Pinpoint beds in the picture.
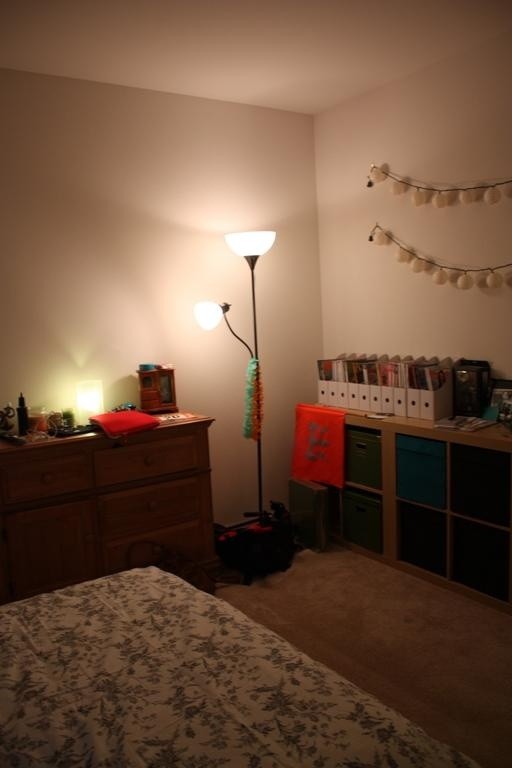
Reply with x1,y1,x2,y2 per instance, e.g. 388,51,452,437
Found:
1,564,480,767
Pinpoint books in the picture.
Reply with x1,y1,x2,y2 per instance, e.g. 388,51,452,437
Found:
317,358,512,432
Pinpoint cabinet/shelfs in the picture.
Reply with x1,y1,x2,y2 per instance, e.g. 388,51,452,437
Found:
312,403,512,615
0,410,216,601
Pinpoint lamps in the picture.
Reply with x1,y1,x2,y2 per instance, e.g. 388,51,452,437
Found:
195,228,276,518
